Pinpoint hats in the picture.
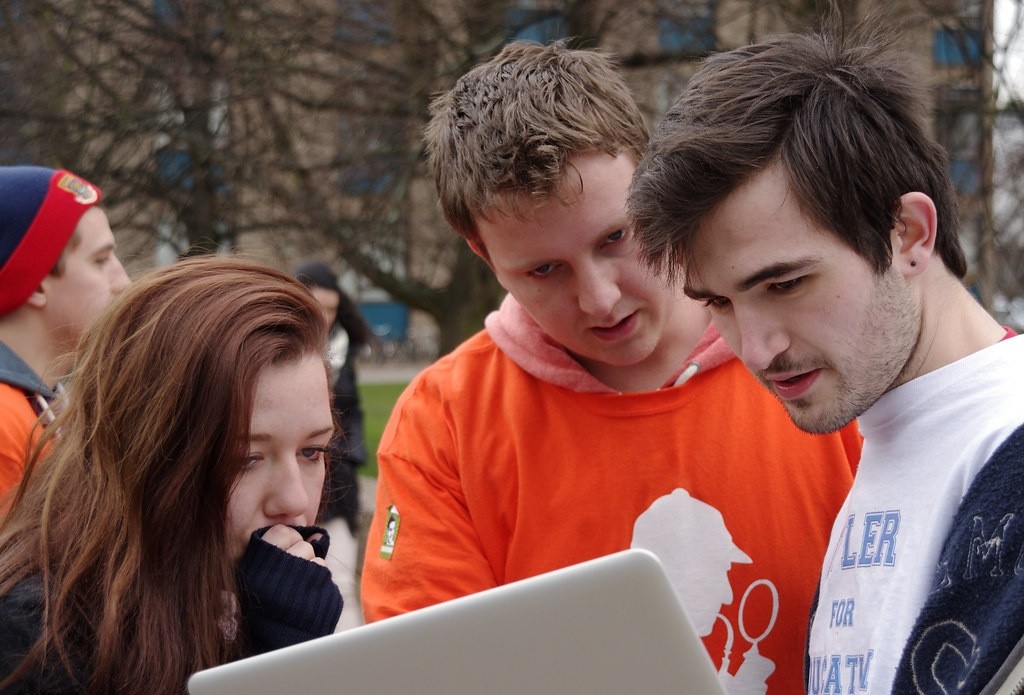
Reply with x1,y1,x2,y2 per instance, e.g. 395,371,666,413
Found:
0,164,104,317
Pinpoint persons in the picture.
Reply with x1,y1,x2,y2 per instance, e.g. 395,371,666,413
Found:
0,160,133,513
0,250,344,694
286,264,366,635
625,0,1024,695
355,43,862,695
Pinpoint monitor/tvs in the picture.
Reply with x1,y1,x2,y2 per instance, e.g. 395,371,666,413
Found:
186,546,728,695
